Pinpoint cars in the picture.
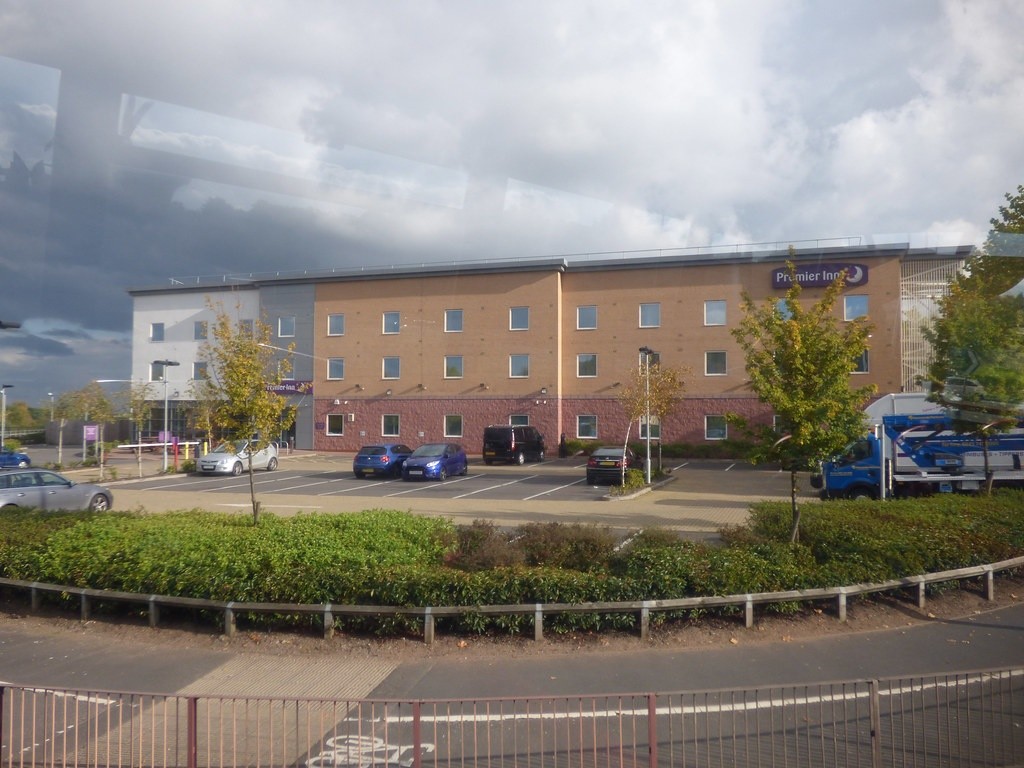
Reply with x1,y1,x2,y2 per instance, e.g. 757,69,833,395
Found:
402,441,468,482
0,468,114,516
0,446,31,470
195,439,279,478
353,442,414,480
587,444,643,486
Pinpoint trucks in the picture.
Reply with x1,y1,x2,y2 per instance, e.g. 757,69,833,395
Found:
854,392,954,427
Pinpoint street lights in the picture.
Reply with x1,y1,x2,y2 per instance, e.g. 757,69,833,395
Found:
1,384,15,446
47,392,54,422
639,346,653,484
153,359,181,472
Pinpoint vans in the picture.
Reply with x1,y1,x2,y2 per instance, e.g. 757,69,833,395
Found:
483,425,545,466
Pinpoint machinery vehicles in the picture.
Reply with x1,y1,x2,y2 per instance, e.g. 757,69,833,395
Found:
810,414,1024,503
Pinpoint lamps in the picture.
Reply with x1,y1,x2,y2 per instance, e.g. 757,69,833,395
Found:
417,383,423,388
355,384,362,388
541,388,547,394
386,389,392,395
334,399,340,405
480,382,486,388
